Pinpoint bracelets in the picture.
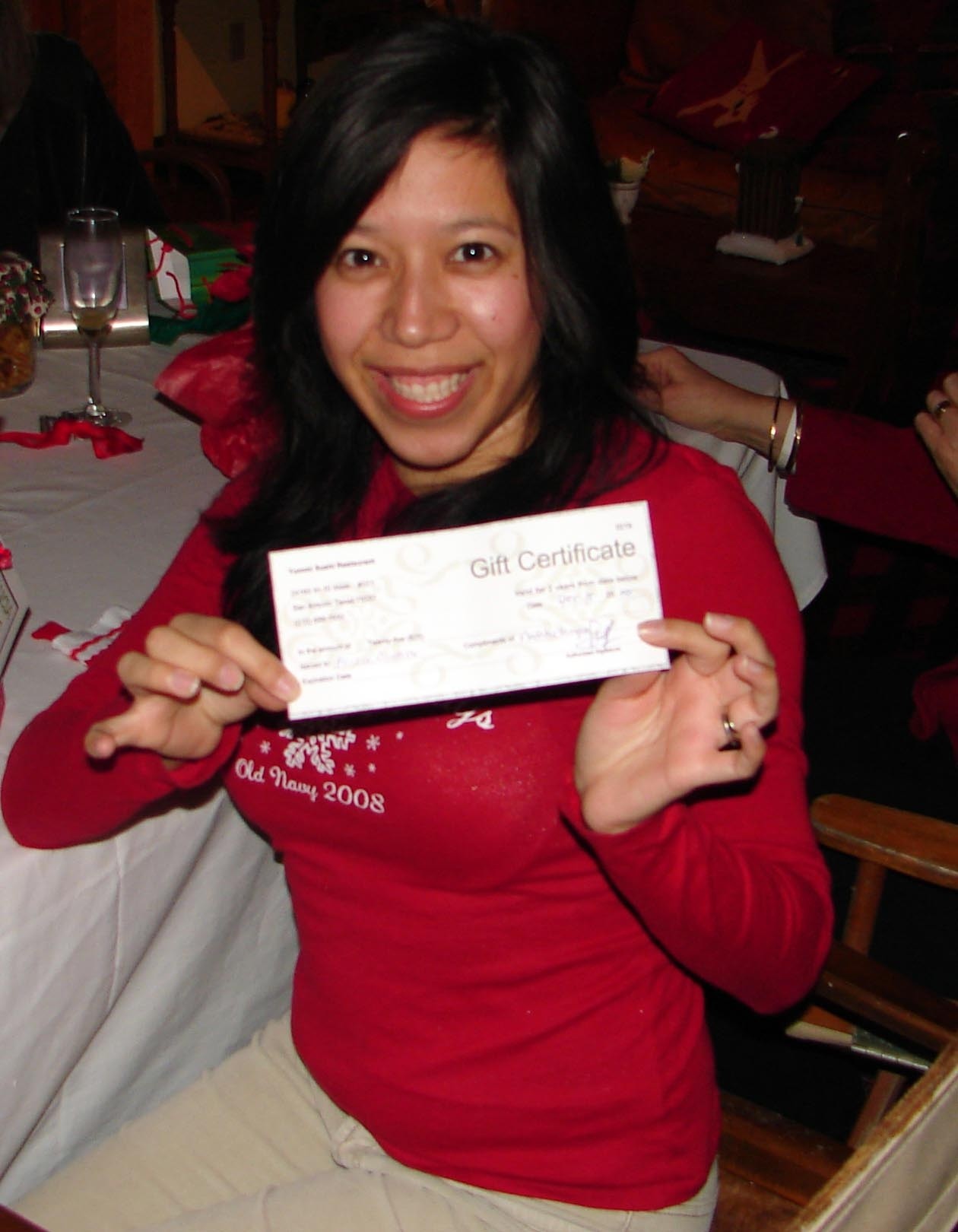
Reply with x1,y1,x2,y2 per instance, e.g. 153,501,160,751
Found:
768,397,781,472
778,402,803,479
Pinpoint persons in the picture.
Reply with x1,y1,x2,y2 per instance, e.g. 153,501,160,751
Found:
626,343,958,762
0,22,835,1232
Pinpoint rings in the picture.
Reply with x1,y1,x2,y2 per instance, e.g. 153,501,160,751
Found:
935,400,950,419
724,714,735,736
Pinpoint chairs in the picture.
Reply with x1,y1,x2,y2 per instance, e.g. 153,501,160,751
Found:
701,794,958,1232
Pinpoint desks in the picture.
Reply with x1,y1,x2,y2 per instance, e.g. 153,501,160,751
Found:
0,338,830,1204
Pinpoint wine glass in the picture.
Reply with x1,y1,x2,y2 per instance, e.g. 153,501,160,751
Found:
610,181,638,223
61,206,135,429
